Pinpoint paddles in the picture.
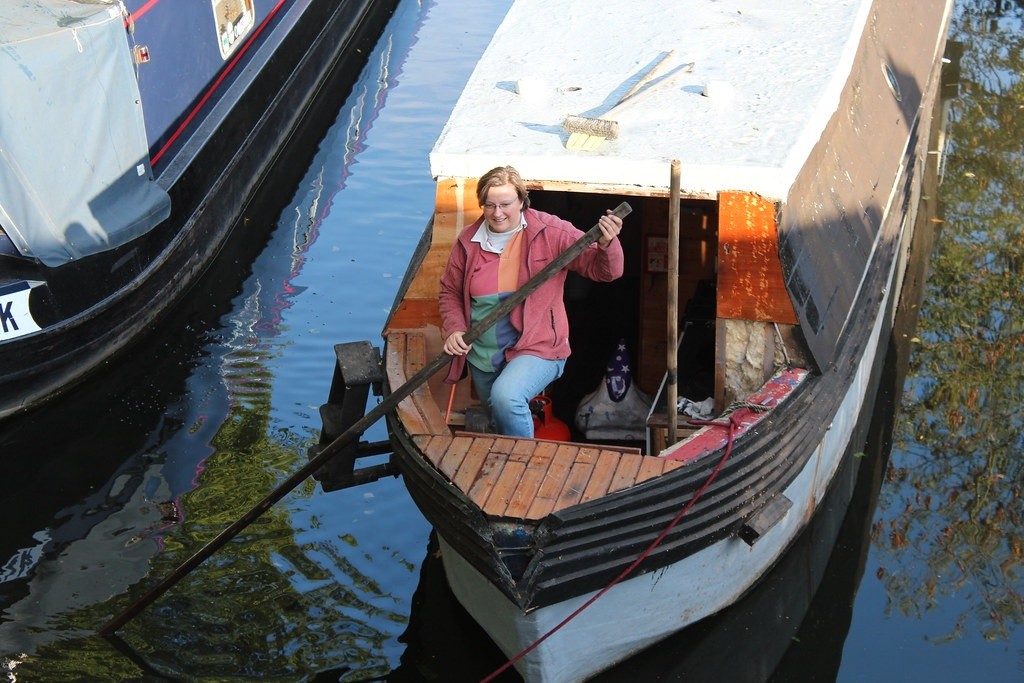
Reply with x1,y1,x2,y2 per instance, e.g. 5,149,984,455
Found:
101,200,633,632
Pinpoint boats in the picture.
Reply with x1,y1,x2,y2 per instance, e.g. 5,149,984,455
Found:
0,1,390,430
363,0,956,683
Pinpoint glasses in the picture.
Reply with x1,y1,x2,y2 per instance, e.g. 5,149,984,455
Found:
479,195,518,210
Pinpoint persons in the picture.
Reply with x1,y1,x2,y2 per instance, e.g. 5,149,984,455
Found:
438,167,624,438
